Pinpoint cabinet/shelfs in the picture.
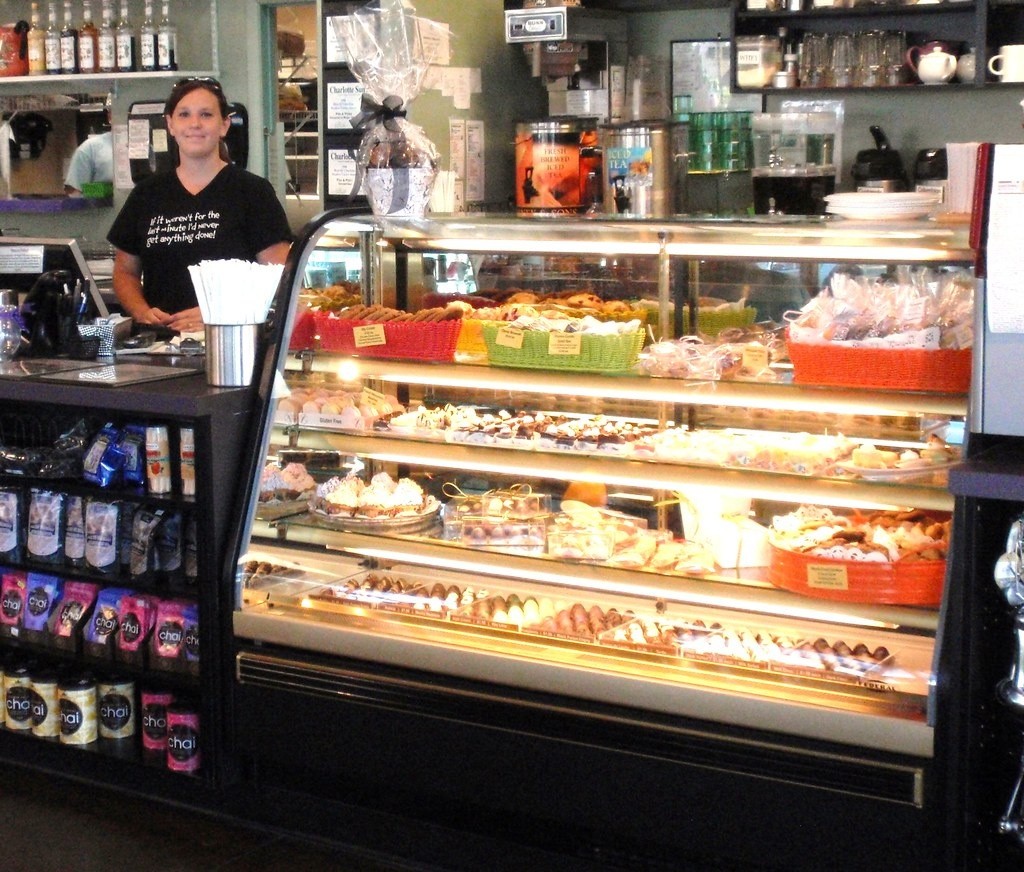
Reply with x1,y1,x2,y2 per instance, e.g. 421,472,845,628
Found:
728,0,1024,95
219,202,985,872
0,473,206,776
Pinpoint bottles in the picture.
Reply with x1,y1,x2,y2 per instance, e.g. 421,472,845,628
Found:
914,147,947,203
138,0,158,71
157,0,178,71
61,0,78,74
115,0,134,72
78,0,99,73
45,2,61,75
179,419,196,496
26,3,47,76
0,305,21,375
98,0,118,73
772,25,806,89
145,417,172,495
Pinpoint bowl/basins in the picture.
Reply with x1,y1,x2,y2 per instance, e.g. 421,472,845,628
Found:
81,240,119,261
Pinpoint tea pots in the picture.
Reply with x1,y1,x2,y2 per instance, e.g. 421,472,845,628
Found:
906,39,976,85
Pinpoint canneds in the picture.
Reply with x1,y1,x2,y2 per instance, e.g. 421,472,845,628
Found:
0,663,201,772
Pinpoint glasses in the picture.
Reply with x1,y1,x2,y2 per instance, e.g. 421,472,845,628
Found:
172,76,223,93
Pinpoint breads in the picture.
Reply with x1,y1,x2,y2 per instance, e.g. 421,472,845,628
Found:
442,300,569,321
503,293,631,313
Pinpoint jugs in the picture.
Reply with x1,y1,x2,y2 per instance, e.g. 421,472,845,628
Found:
0,20,30,77
850,125,909,193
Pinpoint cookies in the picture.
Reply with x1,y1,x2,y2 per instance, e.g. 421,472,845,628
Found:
340,304,465,325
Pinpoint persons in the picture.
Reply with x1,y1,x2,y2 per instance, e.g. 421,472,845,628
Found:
107,75,293,333
64,111,114,195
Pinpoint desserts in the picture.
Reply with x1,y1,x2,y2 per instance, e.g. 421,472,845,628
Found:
238,385,961,685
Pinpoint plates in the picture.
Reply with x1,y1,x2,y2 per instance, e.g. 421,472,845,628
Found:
824,192,938,222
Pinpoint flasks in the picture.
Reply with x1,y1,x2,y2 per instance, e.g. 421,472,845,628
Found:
0,288,19,304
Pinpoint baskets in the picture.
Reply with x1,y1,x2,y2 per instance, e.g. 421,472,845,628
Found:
313,308,462,362
482,319,646,373
650,306,758,342
456,315,513,355
767,536,946,606
783,326,971,393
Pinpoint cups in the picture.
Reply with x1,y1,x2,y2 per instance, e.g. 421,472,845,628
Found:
204,324,265,387
55,313,88,357
609,55,650,120
988,44,1024,82
802,31,908,86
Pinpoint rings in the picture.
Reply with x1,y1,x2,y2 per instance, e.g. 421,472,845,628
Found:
188,322,192,328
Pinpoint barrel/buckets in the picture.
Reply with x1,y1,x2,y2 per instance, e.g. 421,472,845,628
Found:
599,118,673,221
514,114,602,219
